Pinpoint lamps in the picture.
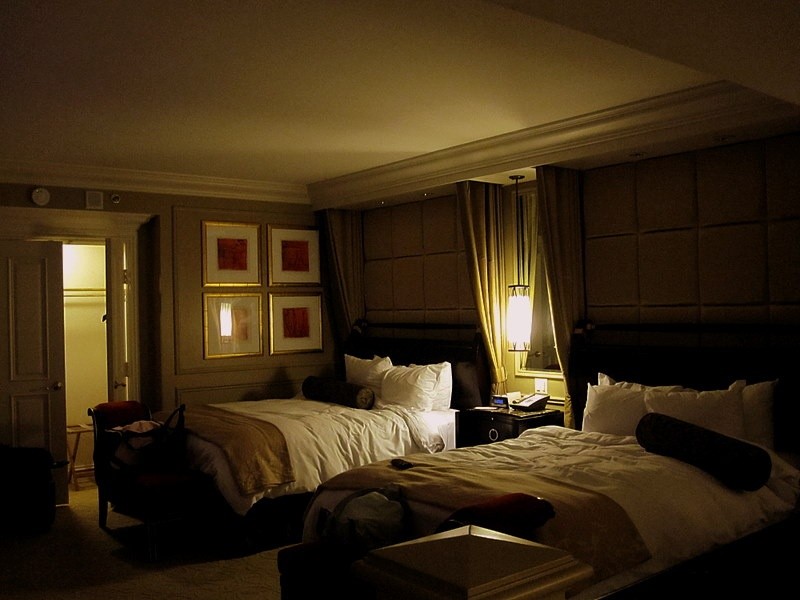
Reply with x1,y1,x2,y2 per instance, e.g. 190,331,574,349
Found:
508,175,533,352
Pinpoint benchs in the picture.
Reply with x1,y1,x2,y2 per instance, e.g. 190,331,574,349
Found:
279,494,556,600
88,400,194,536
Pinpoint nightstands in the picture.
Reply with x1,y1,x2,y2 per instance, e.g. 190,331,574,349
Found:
455,406,564,448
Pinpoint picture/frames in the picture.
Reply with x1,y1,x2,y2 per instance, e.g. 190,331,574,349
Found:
203,292,263,360
266,223,323,287
202,220,263,287
268,292,325,356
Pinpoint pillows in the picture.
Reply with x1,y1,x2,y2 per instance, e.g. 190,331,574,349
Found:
301,354,453,409
580,372,780,492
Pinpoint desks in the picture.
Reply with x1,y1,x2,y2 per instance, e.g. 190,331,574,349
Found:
67,423,94,491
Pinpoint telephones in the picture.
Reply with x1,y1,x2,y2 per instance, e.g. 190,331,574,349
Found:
510,393,550,412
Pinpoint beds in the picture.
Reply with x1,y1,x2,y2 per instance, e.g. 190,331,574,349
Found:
151,323,491,564
301,319,800,600
